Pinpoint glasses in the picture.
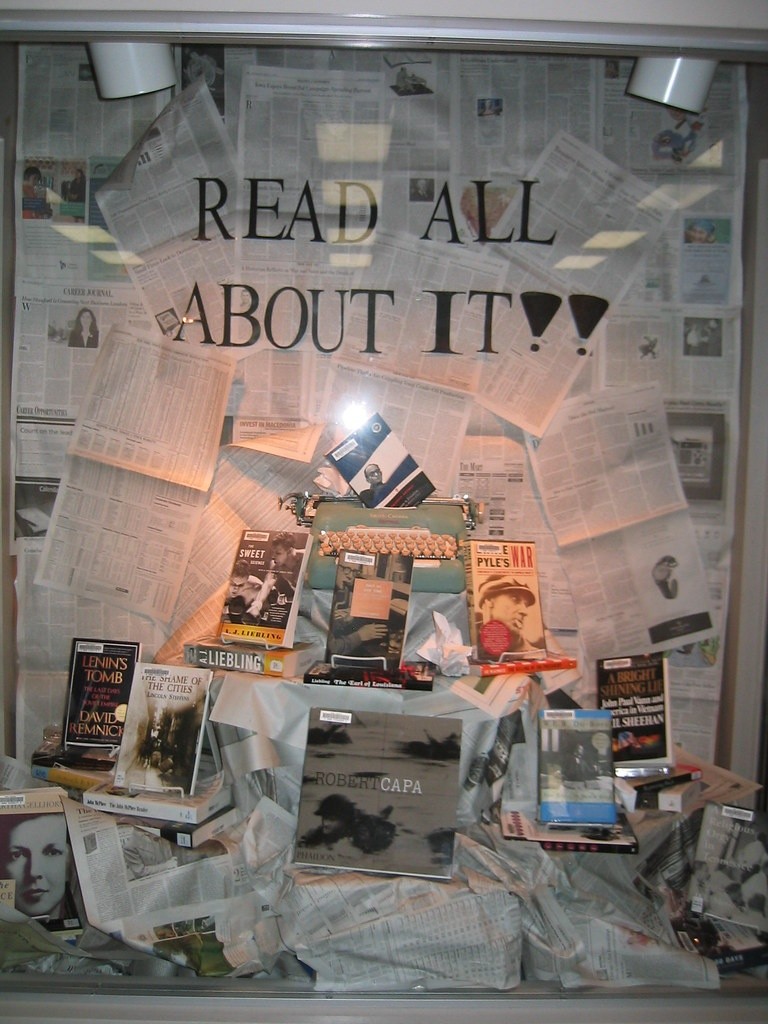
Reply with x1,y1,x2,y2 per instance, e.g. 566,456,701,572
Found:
339,563,361,578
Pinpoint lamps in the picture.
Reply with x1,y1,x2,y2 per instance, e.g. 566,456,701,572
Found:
626,58,722,115
90,42,179,102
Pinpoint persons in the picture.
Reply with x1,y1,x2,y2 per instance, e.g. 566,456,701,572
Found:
0,812,84,933
326,550,389,666
475,572,541,662
222,561,270,629
564,743,597,782
359,463,393,508
240,531,306,626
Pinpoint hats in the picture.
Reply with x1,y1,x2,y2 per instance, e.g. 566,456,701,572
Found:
478,572,535,608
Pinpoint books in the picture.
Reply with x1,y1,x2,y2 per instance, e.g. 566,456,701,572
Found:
302,547,440,693
180,528,328,681
499,708,641,857
594,649,705,816
667,799,768,976
290,705,464,887
0,786,84,946
30,636,239,852
460,538,580,678
324,410,437,511
